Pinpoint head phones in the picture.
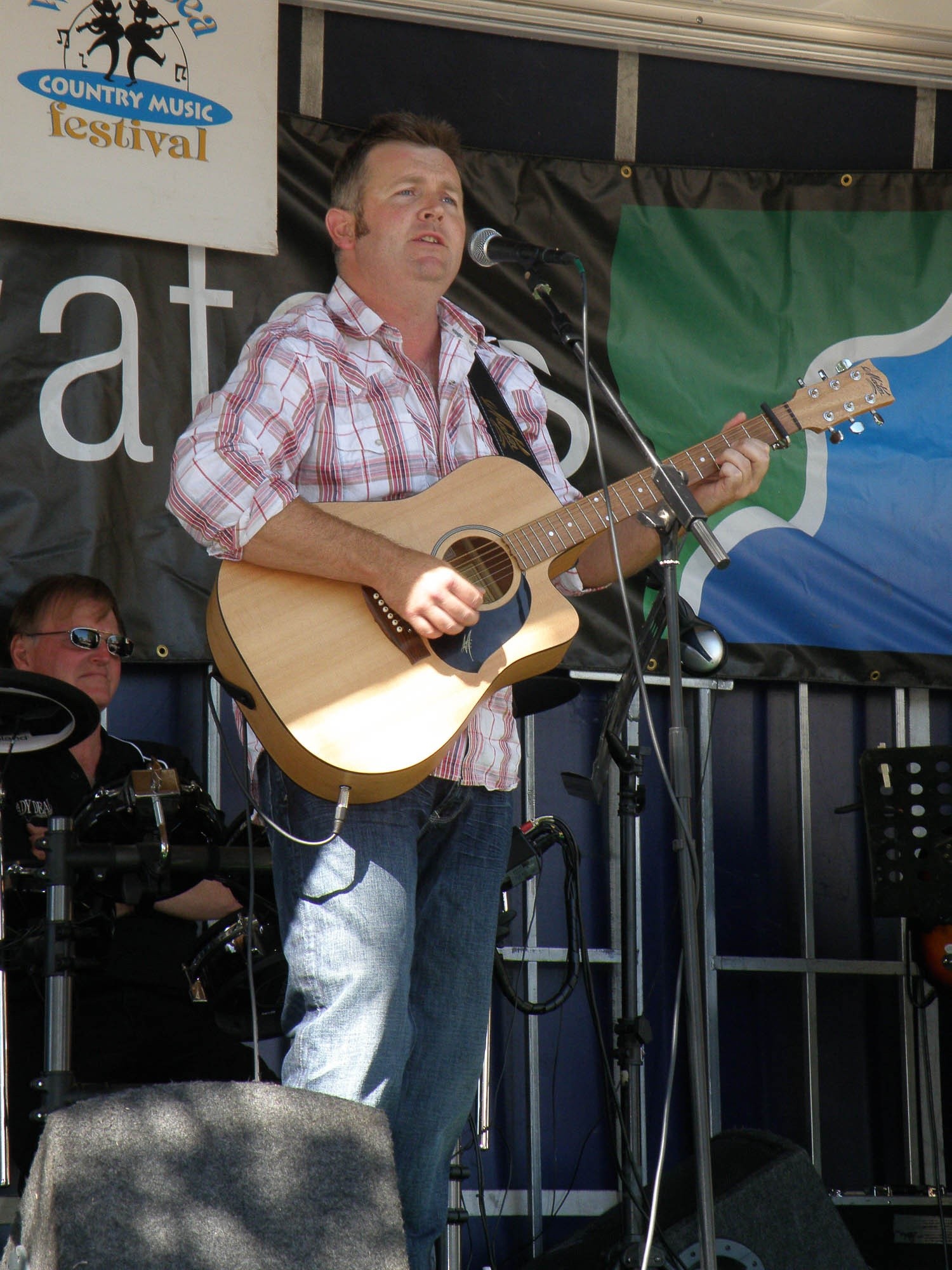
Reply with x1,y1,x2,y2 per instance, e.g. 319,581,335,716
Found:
645,559,730,675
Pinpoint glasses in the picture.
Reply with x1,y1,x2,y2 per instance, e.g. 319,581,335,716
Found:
23,627,135,658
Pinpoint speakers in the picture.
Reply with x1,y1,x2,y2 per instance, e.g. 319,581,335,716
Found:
578,1129,873,1270
2,1082,412,1270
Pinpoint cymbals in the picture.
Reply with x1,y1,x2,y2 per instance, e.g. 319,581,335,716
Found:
0,667,101,760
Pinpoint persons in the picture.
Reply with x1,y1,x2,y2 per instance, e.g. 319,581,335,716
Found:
166,110,773,1270
1,572,256,1090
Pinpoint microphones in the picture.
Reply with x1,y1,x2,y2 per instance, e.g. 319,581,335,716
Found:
466,227,580,267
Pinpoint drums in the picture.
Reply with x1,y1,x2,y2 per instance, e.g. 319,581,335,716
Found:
68,778,221,904
183,903,287,1041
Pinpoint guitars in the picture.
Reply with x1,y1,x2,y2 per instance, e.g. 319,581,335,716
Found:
205,358,896,804
